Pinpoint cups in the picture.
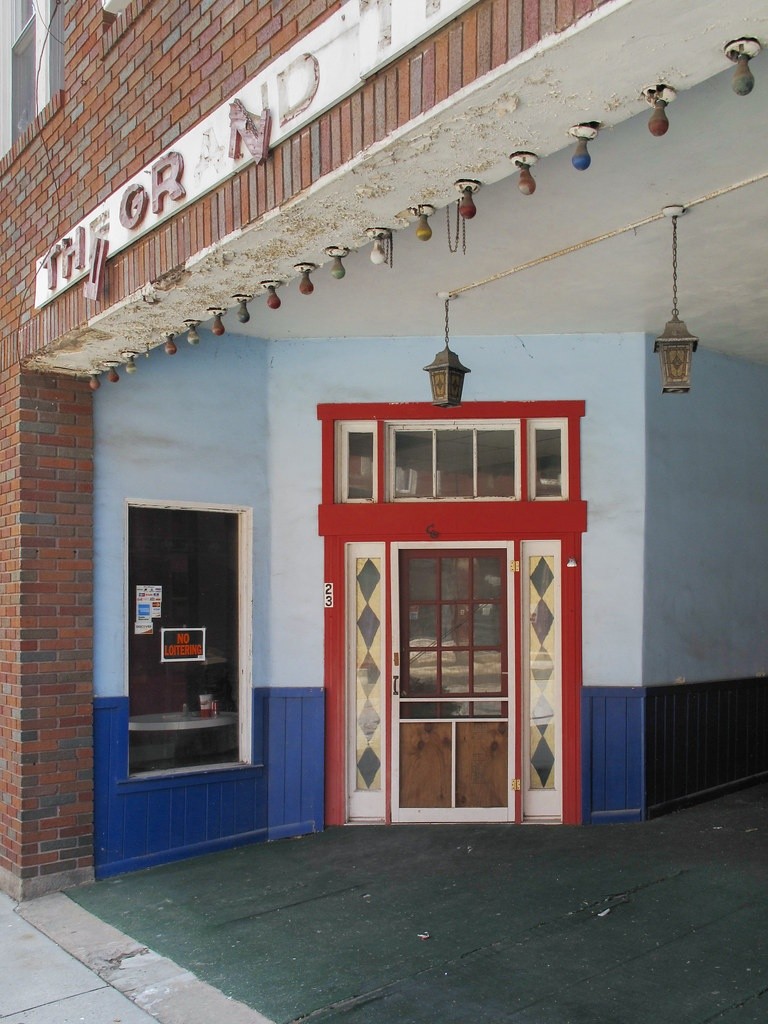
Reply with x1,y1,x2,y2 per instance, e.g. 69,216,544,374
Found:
199,694,213,718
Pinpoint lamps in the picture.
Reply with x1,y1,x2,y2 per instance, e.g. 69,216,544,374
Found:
422,290,472,408
653,205,699,394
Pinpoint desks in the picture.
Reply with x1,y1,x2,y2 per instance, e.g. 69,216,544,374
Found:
129,711,238,770
